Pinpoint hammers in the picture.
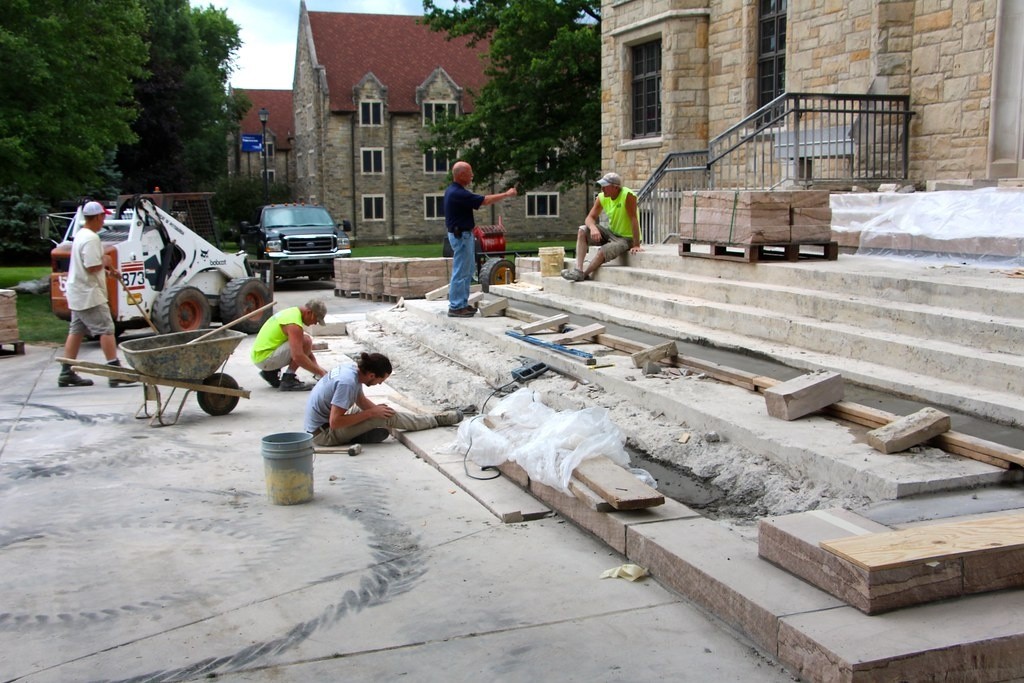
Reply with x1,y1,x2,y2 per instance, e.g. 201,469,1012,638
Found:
313,444,362,456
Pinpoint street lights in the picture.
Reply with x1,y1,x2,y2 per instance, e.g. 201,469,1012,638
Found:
257,107,270,200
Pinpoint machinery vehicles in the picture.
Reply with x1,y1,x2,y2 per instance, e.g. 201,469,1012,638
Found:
39,186,275,342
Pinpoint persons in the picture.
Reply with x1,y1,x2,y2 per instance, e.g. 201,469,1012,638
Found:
444,161,518,318
57,202,136,387
562,172,643,282
306,352,463,447
251,297,327,391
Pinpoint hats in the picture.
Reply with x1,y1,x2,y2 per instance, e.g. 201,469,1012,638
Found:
305,299,327,326
83,201,113,215
597,173,621,187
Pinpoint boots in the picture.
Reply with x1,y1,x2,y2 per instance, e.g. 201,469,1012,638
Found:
58,363,94,387
107,360,137,387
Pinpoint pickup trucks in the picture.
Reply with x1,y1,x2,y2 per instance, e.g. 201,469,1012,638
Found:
240,203,353,285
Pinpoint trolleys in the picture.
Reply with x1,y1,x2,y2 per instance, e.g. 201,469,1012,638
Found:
54,326,253,427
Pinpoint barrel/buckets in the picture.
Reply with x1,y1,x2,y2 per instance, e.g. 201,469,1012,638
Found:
260,432,317,504
538,246,565,277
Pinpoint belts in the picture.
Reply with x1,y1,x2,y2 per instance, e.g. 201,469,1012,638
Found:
311,422,330,439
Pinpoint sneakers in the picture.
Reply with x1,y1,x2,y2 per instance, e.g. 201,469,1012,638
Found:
560,267,584,281
447,305,478,317
352,428,389,443
433,410,464,426
259,369,281,388
279,373,312,391
